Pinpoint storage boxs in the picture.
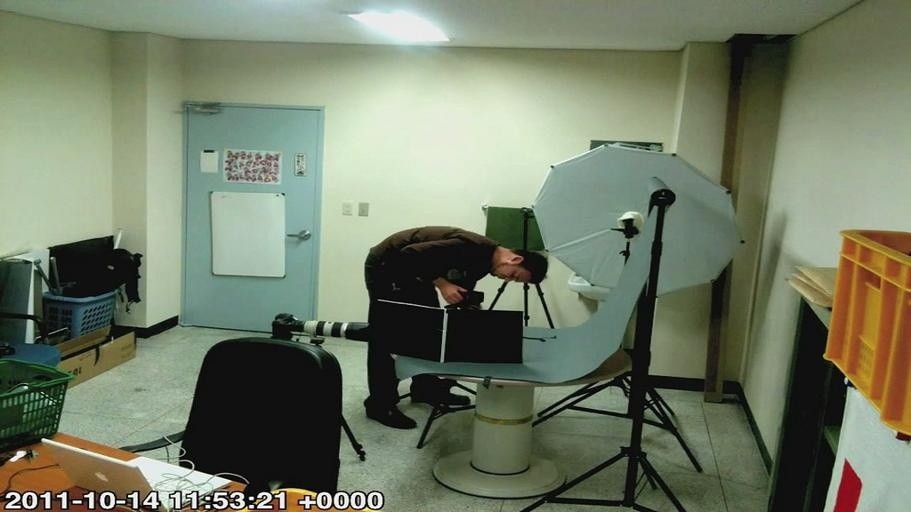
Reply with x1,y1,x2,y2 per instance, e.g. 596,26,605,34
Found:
822,230,911,438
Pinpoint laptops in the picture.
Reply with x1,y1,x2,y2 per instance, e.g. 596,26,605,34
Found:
41,438,233,512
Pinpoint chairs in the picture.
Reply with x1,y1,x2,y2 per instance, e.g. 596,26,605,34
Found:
119,335,345,495
392,175,676,499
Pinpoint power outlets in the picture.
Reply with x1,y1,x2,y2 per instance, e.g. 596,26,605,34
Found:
358,202,369,216
341,201,353,216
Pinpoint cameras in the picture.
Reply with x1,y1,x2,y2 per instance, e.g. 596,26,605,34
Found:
458,290,485,311
272,313,371,344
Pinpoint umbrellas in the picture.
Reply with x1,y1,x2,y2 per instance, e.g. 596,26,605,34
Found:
531,145,745,300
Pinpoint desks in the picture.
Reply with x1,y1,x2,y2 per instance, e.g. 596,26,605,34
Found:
0,432,380,512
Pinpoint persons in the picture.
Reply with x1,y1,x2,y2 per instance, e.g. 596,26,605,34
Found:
364,227,548,429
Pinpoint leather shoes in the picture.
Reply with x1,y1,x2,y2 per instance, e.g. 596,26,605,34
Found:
410,378,472,408
363,399,417,430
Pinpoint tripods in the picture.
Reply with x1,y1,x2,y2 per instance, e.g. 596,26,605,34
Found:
530,207,705,476
537,282,678,433
483,206,555,329
396,373,475,448
519,176,686,512
